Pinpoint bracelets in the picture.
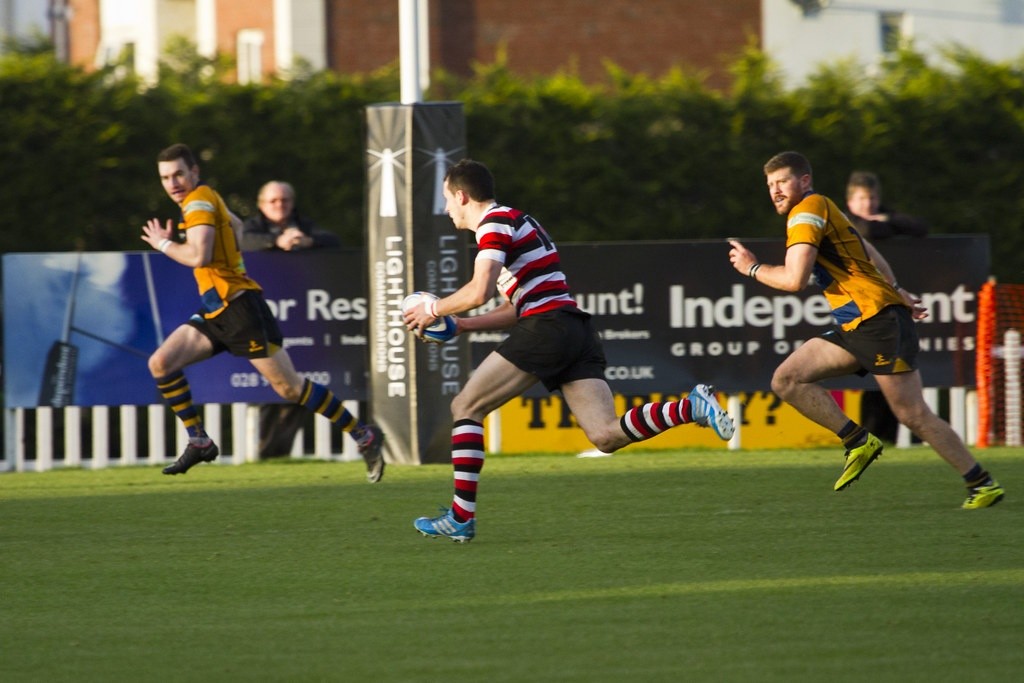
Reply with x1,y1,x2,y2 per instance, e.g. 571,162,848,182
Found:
747,262,762,278
892,283,902,292
155,238,172,254
425,300,439,319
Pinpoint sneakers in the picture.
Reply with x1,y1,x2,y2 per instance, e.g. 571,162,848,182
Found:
162,439,219,475
357,425,385,483
834,432,884,492
414,504,476,543
962,477,1005,509
687,384,735,441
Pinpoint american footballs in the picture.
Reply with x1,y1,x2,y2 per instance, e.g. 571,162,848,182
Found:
399,290,460,345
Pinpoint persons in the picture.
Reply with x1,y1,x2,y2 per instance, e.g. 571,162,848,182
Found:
242,180,342,253
403,159,734,544
843,165,925,239
728,153,1008,511
141,144,387,485
